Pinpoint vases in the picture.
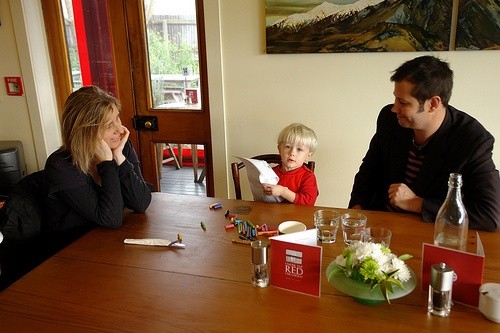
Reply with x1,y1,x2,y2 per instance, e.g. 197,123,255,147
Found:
325,259,416,306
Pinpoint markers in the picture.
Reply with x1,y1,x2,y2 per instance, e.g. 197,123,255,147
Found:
225,209,229,216
200,221,206,230
210,203,222,209
225,214,278,244
178,233,182,243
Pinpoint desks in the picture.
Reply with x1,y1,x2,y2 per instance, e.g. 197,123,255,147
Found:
154,102,204,183
0,196,500,333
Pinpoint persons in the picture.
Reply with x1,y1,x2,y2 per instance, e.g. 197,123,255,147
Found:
265,122,318,207
348,55,500,233
42,85,151,262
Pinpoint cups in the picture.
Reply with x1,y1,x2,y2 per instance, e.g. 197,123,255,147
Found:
277,220,306,235
479,282,500,323
340,211,367,247
313,209,340,243
361,226,392,249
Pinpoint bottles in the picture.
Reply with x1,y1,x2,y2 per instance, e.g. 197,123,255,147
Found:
427,262,455,317
250,240,270,288
434,172,469,252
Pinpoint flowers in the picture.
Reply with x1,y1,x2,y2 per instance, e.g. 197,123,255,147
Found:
333,240,410,292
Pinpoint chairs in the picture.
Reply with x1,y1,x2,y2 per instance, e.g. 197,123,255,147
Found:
230,153,315,202
